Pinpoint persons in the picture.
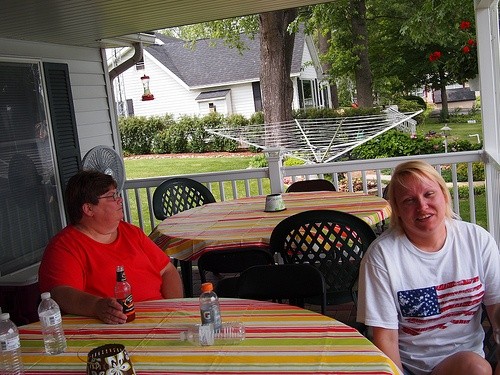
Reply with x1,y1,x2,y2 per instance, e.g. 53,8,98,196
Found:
39,169,183,325
356,160,500,375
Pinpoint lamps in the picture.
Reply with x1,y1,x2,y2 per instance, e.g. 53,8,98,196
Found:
141,62,155,101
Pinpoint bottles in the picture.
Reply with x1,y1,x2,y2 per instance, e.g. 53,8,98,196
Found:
199,283,223,326
0,313,23,375
37,292,68,356
180,321,246,346
114,265,136,323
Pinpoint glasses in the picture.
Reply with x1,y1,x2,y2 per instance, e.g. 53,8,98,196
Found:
98,191,122,200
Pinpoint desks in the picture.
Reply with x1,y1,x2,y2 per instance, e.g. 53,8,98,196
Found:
149,190,393,298
0,297,407,375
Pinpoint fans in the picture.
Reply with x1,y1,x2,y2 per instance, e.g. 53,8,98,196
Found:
8,155,55,243
81,146,126,195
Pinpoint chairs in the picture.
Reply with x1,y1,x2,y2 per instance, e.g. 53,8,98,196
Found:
268,209,377,315
197,248,284,305
152,176,217,269
